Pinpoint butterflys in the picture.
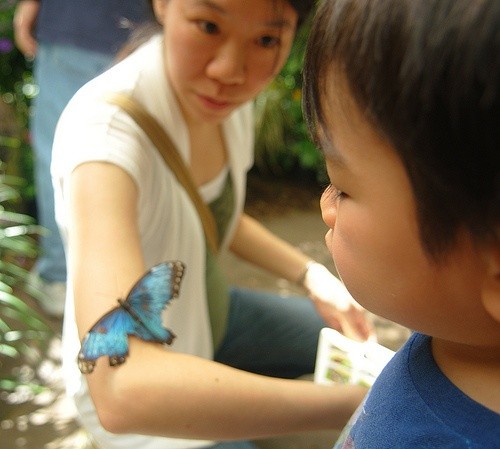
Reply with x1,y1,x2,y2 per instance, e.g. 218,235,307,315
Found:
76,260,185,375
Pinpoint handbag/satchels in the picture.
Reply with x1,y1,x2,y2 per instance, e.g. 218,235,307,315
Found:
209,248,232,350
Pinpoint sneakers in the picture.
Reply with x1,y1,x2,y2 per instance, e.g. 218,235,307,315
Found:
26,259,67,318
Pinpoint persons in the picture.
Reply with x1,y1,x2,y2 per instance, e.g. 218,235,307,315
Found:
13,1,157,318
297,0,500,449
51,0,375,449
